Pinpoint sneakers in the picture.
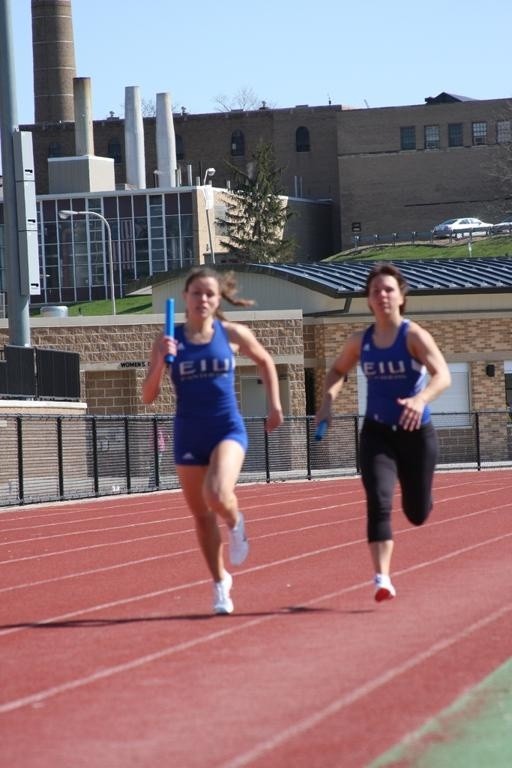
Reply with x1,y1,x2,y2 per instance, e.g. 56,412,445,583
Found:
228,512,249,567
212,568,234,615
375,574,396,601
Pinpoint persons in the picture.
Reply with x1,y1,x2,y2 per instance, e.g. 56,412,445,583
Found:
313,261,455,602
147,421,170,488
136,265,284,617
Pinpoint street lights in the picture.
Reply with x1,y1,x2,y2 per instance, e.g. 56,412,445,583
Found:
59,210,116,315
203,168,216,185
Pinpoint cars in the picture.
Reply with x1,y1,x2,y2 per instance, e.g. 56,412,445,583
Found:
431,218,494,239
491,216,512,234
